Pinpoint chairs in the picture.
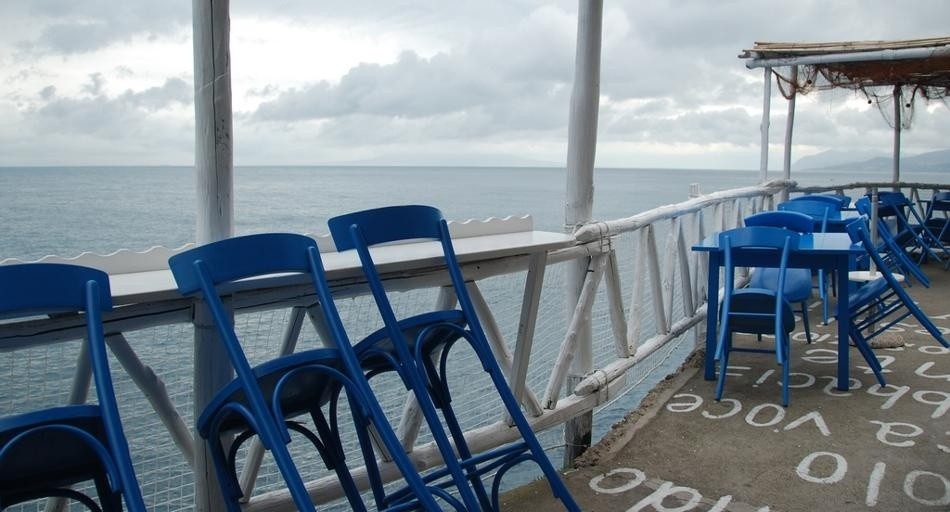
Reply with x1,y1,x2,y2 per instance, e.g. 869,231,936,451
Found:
691,187,950,407
325,198,592,512
163,233,447,512
2,254,159,510
